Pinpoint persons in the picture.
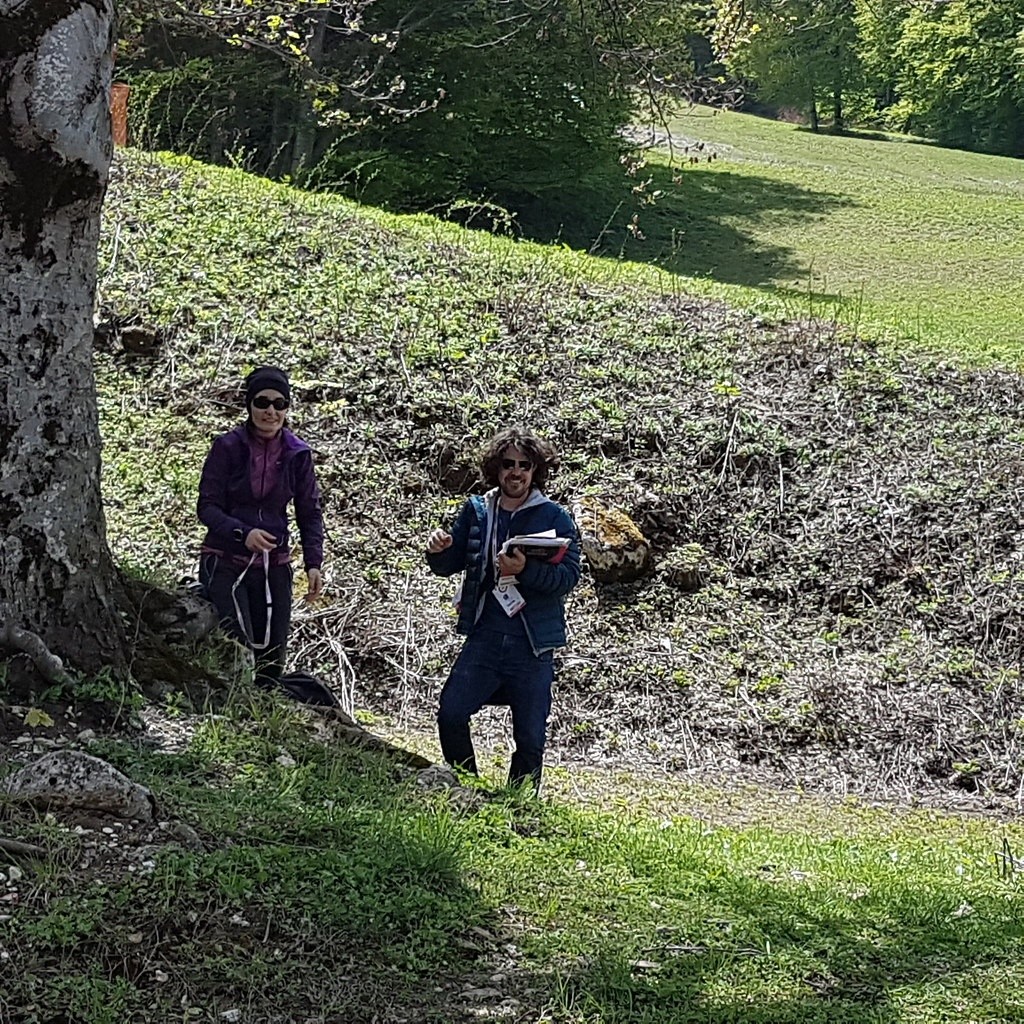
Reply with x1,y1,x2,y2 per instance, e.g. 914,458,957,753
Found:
426,428,584,800
196,365,324,674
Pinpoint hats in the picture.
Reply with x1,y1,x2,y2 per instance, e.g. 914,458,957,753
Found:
245,366,291,408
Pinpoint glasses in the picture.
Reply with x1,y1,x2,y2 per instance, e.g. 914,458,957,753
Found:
501,457,532,472
252,395,290,411
